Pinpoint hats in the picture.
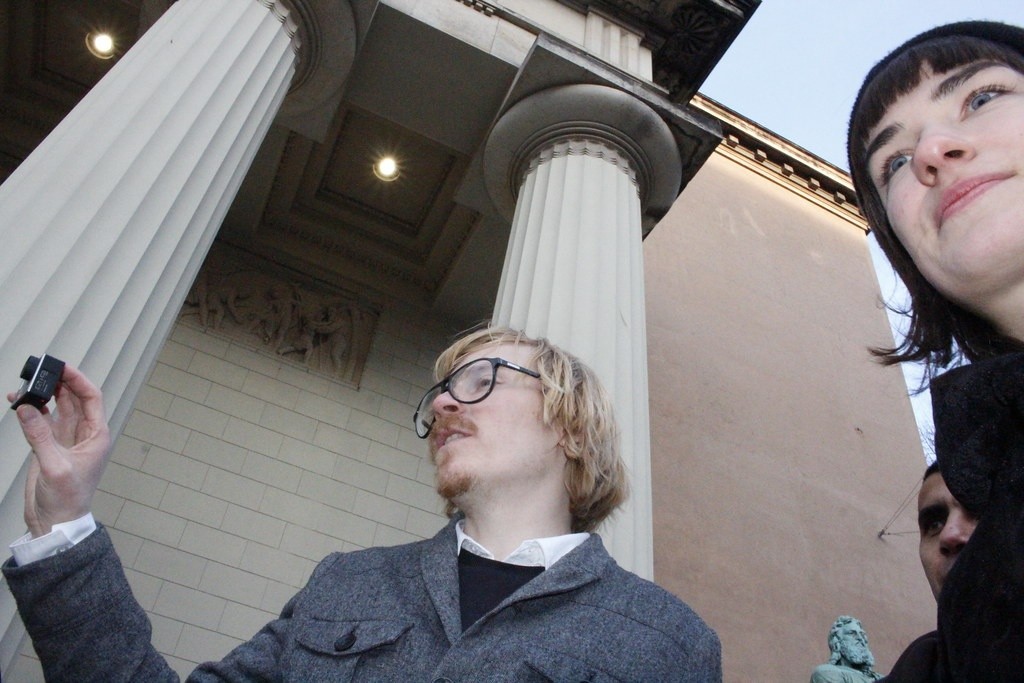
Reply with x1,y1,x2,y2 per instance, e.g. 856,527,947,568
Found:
847,20,1024,219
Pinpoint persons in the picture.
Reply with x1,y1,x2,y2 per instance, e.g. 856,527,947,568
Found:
2,323,725,681
844,73,1021,680
808,614,886,682
887,456,990,682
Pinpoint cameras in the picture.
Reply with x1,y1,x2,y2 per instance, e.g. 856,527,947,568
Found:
11,353,65,410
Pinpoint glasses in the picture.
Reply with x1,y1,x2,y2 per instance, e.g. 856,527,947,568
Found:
412,357,540,439
